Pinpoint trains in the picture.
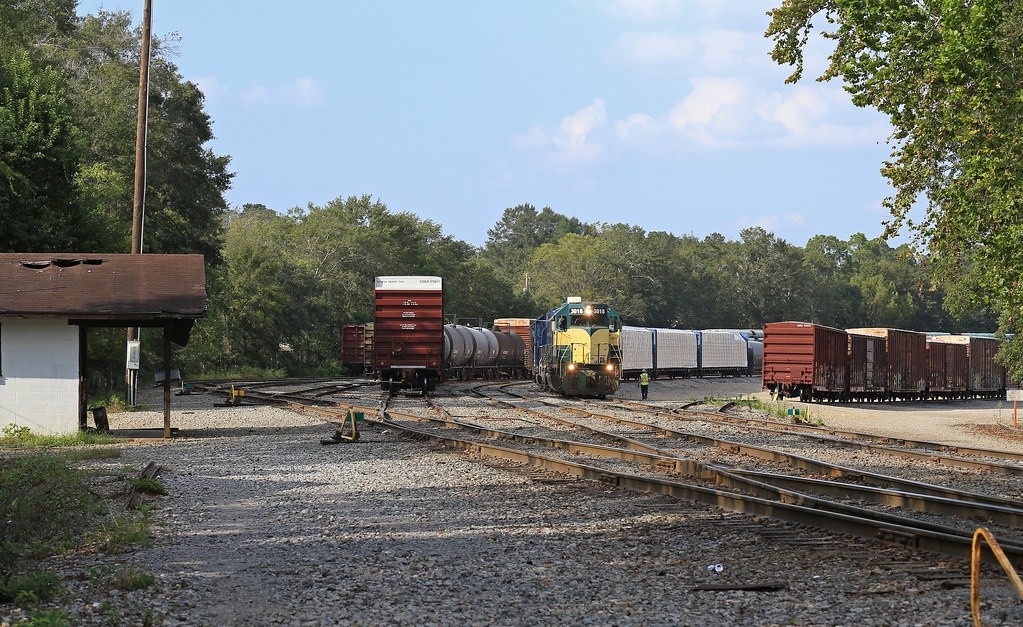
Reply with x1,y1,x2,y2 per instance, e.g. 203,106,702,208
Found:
530,295,762,391
761,319,1023,404
374,273,532,394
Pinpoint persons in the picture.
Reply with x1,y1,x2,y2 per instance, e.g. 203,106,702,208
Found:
638,368,650,400
